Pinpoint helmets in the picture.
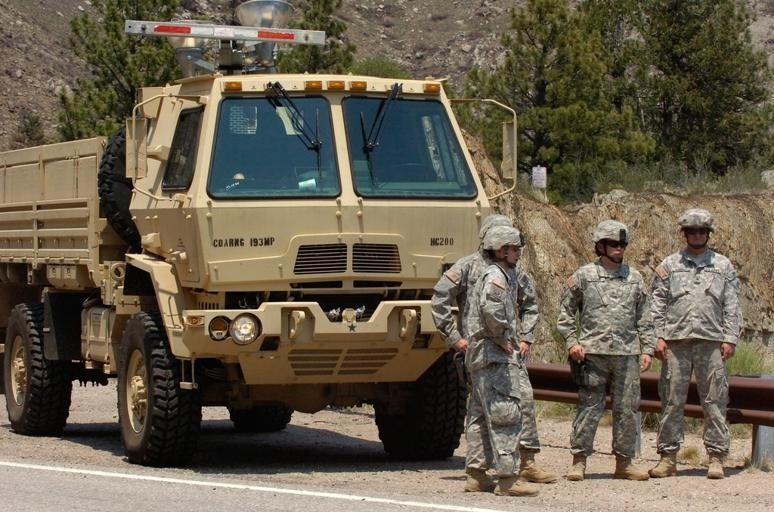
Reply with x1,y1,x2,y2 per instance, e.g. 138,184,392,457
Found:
679,207,715,232
479,214,513,241
483,225,526,253
590,219,629,245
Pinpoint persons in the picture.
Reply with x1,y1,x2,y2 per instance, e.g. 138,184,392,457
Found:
430,214,555,484
556,220,656,482
648,207,741,478
461,224,557,496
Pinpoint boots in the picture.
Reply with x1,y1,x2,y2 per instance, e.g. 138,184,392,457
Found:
517,448,560,484
648,451,680,479
567,453,587,481
493,477,540,497
706,451,726,479
464,470,496,493
613,453,651,481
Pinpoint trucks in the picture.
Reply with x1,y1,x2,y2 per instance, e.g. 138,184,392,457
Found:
0,1,518,460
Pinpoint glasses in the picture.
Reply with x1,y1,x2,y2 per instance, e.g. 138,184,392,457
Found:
606,239,627,249
